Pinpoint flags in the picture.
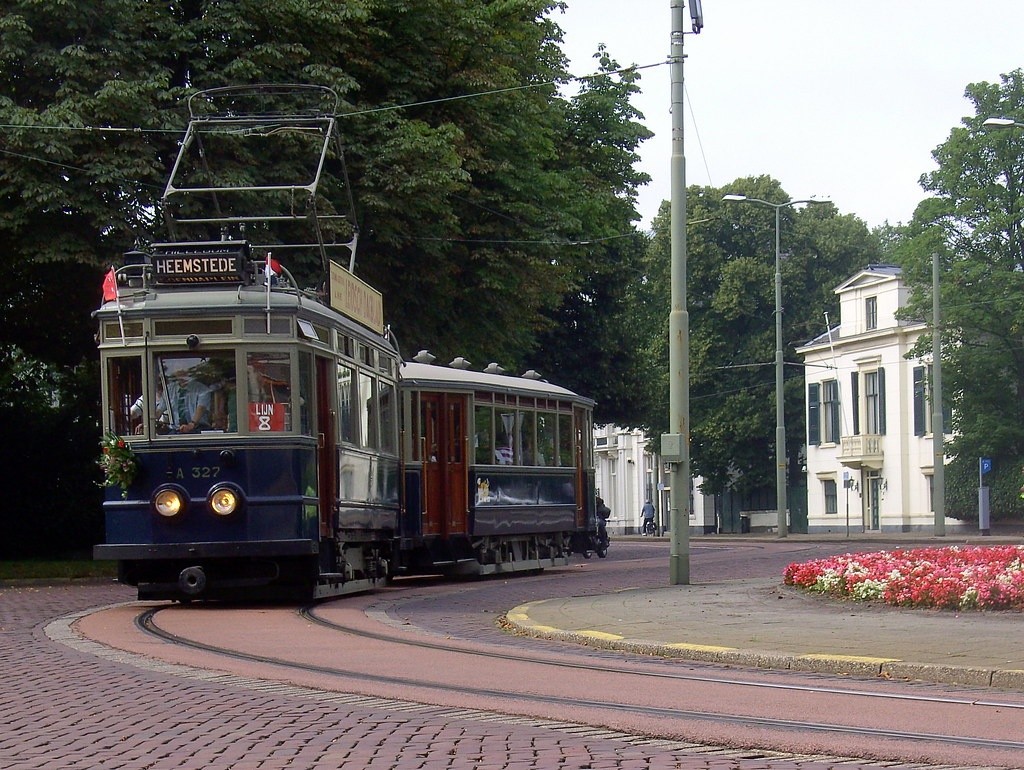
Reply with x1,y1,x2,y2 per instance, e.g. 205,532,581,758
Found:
102,270,116,300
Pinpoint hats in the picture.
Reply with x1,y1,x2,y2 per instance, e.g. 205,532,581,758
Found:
645,499,650,502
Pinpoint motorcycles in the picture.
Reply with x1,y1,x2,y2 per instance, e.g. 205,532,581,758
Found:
582,520,611,559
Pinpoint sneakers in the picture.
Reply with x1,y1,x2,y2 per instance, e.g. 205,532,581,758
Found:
642,533,647,536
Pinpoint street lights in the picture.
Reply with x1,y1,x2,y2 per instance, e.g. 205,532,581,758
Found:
722,194,833,537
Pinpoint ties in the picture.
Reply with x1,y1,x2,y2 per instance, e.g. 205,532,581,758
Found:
178,389,188,426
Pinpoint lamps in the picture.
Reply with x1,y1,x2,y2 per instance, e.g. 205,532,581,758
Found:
627,457,633,465
878,474,887,491
849,476,858,492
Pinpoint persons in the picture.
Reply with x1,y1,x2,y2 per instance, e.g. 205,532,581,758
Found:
496,433,513,465
475,432,495,465
524,436,546,466
129,362,213,434
640,499,655,536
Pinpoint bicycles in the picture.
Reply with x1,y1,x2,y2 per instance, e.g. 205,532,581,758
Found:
638,517,657,539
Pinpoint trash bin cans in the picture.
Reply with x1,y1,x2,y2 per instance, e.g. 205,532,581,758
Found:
739,515,750,534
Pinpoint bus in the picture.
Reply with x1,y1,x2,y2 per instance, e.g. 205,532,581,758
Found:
89,236,598,612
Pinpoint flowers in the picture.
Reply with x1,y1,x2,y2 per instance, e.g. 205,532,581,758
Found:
91,429,139,501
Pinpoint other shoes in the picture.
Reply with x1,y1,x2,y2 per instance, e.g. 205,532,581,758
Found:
604,541,610,546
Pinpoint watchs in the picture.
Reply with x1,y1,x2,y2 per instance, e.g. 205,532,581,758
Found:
190,421,196,429
